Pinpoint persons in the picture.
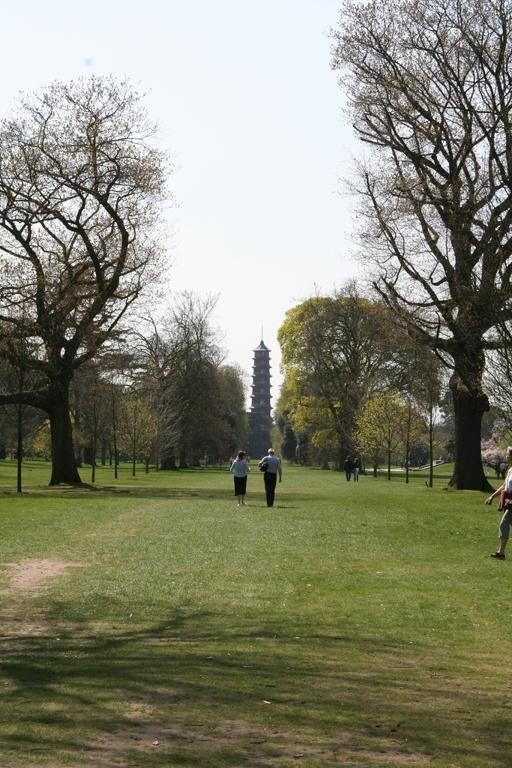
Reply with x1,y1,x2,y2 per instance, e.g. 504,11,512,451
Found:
343,456,352,481
353,458,359,482
485,446,512,560
230,451,250,466
258,448,282,507
230,451,250,507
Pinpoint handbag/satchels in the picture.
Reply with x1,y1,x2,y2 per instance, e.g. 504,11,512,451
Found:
497,489,512,512
259,462,269,472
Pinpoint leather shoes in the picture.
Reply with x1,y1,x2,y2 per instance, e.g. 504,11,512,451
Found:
491,551,505,559
